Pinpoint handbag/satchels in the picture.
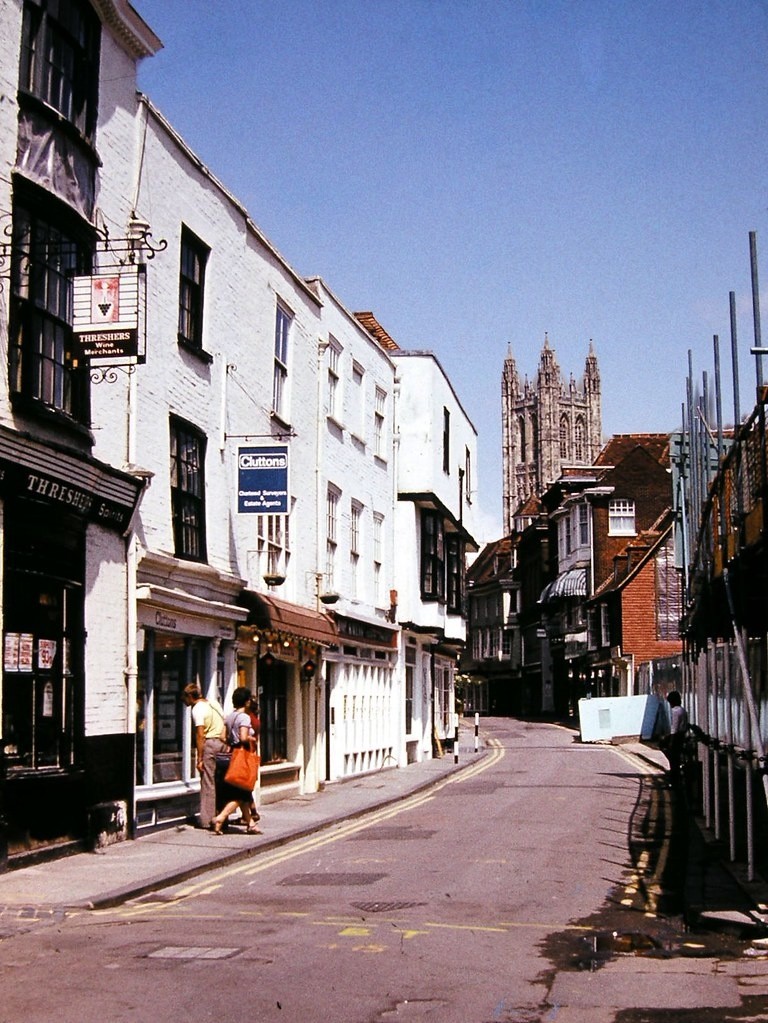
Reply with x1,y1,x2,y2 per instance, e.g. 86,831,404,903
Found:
224,742,261,791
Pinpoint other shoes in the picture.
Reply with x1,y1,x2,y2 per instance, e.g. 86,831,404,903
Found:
195,813,263,834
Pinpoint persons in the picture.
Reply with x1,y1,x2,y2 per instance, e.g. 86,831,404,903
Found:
666,688,690,784
179,682,265,836
